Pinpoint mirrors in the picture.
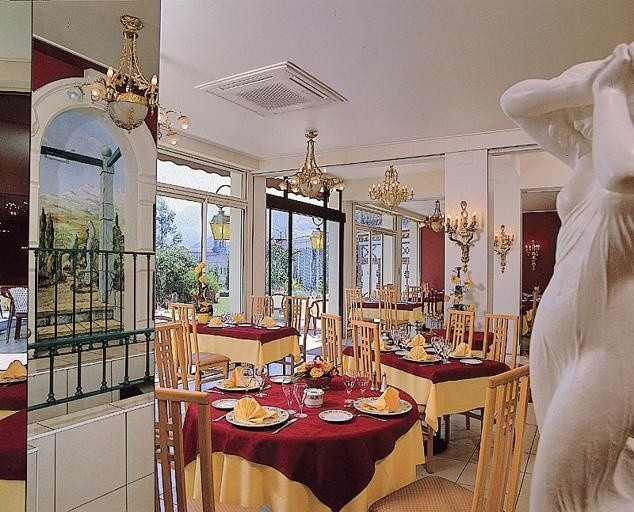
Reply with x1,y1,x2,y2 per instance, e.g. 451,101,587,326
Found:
0,0,34,510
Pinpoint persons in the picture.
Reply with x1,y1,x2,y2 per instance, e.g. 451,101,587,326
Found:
497,41,634,512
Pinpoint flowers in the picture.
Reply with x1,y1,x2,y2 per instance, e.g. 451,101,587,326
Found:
184,261,220,313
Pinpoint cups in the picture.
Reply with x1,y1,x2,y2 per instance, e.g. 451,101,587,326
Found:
303,387,325,408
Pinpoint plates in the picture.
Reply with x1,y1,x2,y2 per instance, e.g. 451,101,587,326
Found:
459,358,483,364
215,378,265,393
204,310,281,330
270,375,294,382
226,406,289,429
352,396,415,416
318,409,353,422
210,399,239,409
367,345,398,352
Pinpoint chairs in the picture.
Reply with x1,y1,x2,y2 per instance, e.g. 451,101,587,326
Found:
0,283,27,344
153,282,540,474
366,361,537,512
150,386,265,511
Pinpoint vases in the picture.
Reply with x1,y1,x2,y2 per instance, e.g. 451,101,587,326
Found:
197,313,208,324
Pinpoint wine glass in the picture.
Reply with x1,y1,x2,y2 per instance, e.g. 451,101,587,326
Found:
281,376,296,416
254,365,268,397
342,368,357,404
391,325,455,364
292,382,308,418
241,368,254,395
356,370,371,401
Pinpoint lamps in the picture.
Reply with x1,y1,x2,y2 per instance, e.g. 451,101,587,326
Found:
418,197,447,234
442,200,482,265
275,127,347,202
524,238,541,273
307,217,329,254
66,10,191,151
367,164,415,212
491,222,515,274
207,184,232,243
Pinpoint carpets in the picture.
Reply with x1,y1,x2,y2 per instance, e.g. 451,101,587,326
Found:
0,318,27,354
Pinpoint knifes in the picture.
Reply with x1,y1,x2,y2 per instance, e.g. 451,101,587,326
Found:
418,363,437,366
271,418,299,436
357,413,388,422
205,389,236,396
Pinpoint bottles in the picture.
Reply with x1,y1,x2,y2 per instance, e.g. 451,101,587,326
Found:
378,373,390,393
371,370,378,391
